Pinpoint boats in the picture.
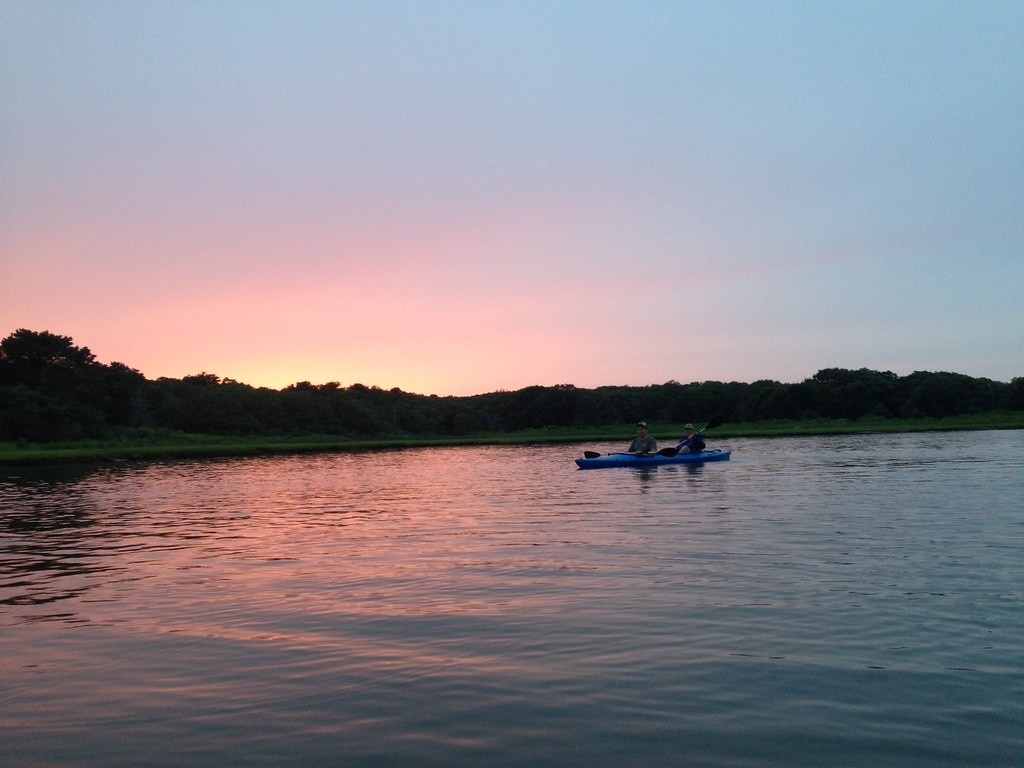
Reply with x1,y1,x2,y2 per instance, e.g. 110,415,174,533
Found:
574,447,733,470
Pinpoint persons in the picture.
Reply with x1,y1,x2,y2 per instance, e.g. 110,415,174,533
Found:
676,424,706,454
624,422,657,456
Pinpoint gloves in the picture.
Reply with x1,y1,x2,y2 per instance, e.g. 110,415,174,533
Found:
688,435,693,439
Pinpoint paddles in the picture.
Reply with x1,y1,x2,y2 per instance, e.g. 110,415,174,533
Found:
583,447,676,459
676,415,725,450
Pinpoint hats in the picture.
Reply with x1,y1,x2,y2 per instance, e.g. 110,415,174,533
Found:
636,422,647,429
684,424,694,429
